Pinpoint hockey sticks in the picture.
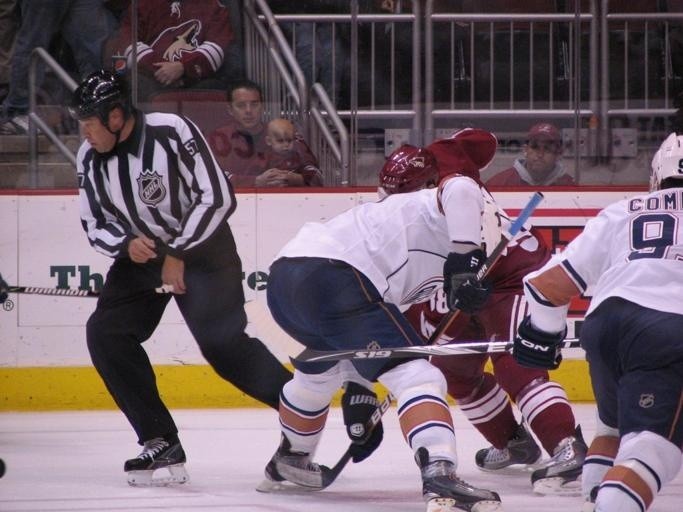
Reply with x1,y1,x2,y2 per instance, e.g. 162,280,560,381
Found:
276,187,543,491
286,340,585,365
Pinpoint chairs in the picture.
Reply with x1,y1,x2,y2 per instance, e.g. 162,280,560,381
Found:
140,0,681,192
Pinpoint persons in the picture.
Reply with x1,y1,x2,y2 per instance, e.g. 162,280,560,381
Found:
2,0,243,138
486,122,577,187
512,132,683,511
265,171,500,509
64,68,295,469
378,129,590,486
245,117,302,187
204,76,325,188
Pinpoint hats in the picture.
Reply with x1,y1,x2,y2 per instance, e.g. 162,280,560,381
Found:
525,122,562,148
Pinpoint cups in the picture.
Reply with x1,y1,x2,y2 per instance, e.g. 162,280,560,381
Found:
111,57,127,76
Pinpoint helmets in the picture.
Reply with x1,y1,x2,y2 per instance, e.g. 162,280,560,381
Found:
68,72,130,121
648,133,682,189
378,144,437,196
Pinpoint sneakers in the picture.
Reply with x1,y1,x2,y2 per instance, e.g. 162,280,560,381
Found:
123,434,187,472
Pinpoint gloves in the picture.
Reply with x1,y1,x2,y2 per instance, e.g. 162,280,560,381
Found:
341,382,385,464
511,315,569,370
443,242,489,313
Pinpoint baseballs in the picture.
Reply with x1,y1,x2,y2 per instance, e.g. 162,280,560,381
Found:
151,468,172,488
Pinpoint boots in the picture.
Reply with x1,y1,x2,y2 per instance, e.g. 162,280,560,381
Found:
475,415,540,471
264,431,334,489
413,447,499,502
531,424,588,482
